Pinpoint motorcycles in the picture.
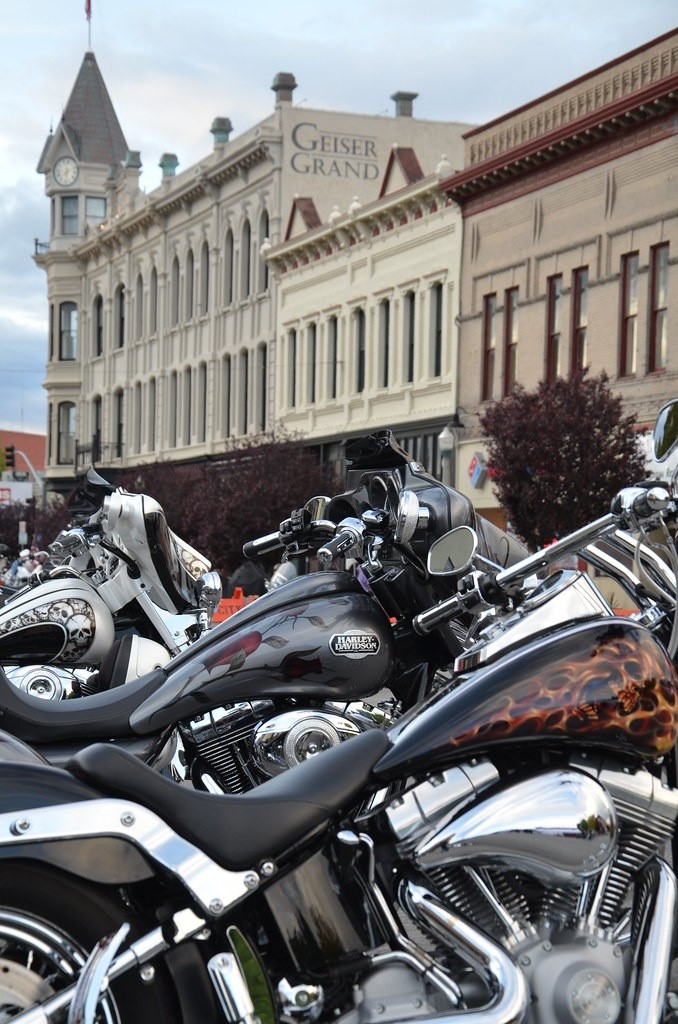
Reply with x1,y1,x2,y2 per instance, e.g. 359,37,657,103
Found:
0,570,676,1024
0,399,677,795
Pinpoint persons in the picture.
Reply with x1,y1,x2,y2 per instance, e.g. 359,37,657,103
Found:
204,556,231,596
0,538,52,590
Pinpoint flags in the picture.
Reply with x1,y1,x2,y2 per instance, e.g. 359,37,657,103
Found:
85,0,92,22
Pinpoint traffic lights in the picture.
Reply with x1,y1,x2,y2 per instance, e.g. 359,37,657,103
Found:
4,445,15,466
